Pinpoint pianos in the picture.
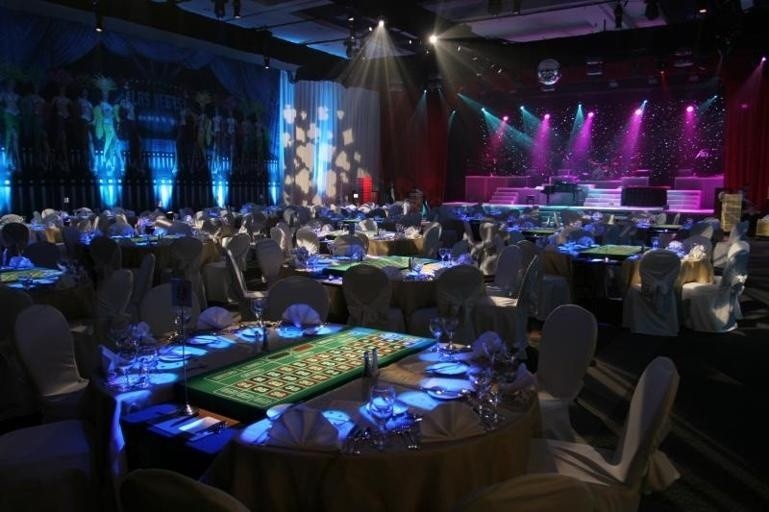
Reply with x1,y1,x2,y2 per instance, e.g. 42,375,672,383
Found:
541,176,582,205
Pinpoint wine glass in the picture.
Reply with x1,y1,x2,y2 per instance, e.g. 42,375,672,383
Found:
250,299,267,329
411,256,423,275
438,247,454,267
442,319,459,352
327,242,336,257
428,317,446,354
116,321,522,455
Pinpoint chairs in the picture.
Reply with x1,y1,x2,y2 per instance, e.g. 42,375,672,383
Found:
214,0,374,70
1,203,751,512
